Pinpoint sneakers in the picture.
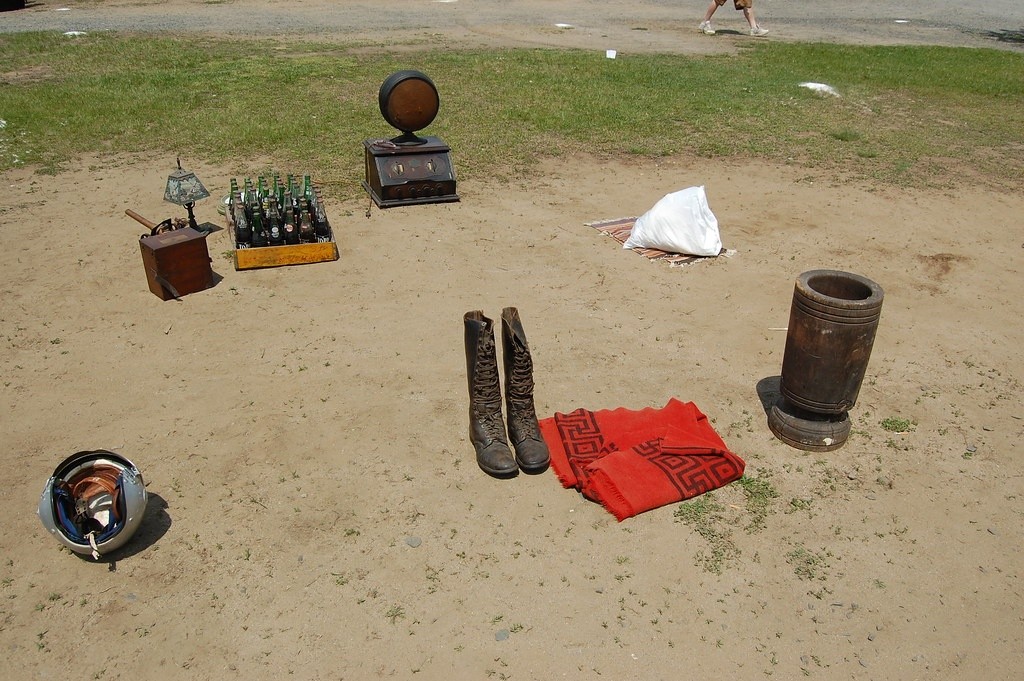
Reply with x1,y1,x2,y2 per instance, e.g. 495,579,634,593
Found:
697,20,716,35
750,25,769,37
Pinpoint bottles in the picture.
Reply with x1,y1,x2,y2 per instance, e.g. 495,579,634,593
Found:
225,174,330,250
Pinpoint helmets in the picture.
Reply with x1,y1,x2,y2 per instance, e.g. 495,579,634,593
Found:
37,449,148,555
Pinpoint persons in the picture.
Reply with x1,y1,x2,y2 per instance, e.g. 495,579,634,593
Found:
697,0,769,37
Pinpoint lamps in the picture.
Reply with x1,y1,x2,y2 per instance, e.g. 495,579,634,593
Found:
162,157,211,232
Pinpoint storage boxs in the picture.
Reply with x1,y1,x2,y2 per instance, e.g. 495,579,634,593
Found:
224,203,340,270
140,226,214,302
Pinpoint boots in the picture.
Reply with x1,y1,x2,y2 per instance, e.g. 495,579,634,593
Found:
501,307,551,471
463,309,520,474
